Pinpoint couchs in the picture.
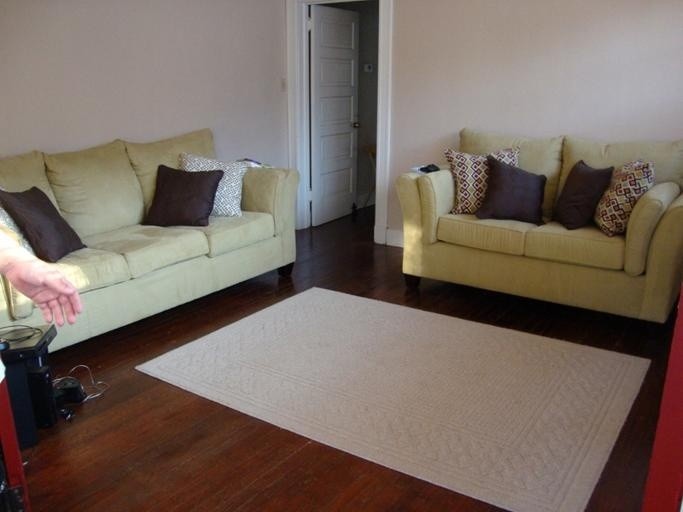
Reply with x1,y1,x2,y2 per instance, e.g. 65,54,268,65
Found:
1,125,302,355
394,124,682,356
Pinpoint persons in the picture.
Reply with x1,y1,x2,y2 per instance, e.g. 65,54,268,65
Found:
1,228,86,329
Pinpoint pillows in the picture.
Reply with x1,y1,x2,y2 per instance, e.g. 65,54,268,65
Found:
435,142,656,239
138,150,250,226
0,185,91,262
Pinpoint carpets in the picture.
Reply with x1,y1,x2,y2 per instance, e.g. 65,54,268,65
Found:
133,282,652,511
